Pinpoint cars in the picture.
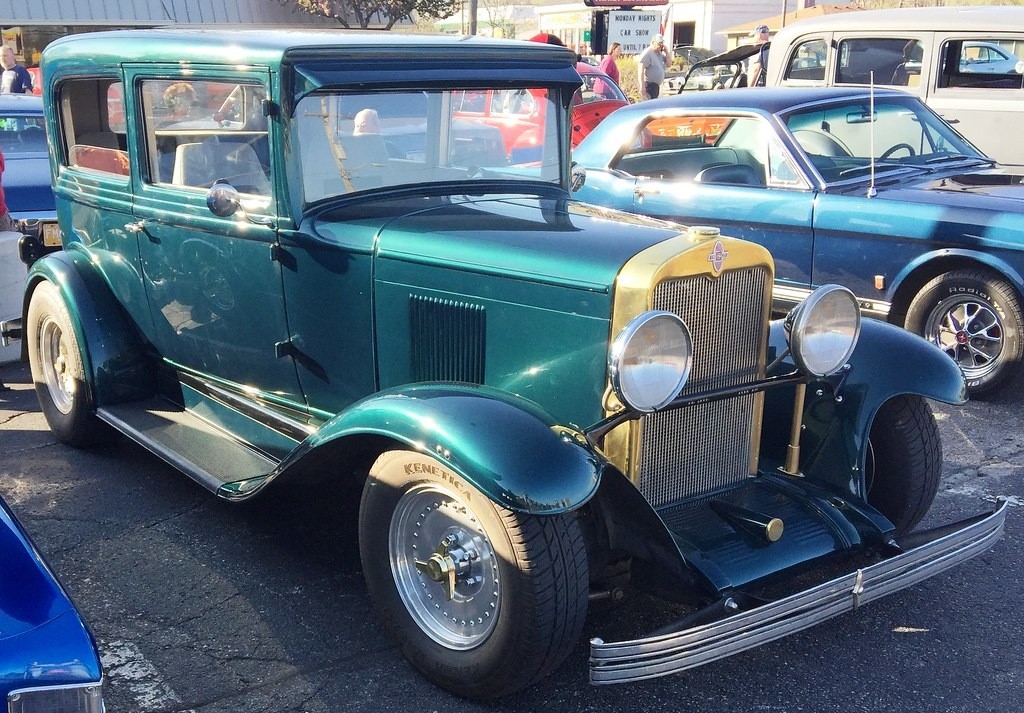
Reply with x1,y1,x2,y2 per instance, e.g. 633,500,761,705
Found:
642,41,773,148
21,26,1017,697
479,83,1024,397
1,88,71,376
448,60,661,172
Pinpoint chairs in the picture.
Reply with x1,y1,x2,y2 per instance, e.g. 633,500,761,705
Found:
173,139,280,202
642,160,769,186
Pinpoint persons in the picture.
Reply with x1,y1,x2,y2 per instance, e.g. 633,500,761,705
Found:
745,25,772,86
161,76,247,129
635,32,671,100
353,108,408,161
1,45,34,96
593,42,621,98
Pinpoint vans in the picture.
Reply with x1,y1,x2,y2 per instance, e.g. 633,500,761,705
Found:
764,5,1024,185
